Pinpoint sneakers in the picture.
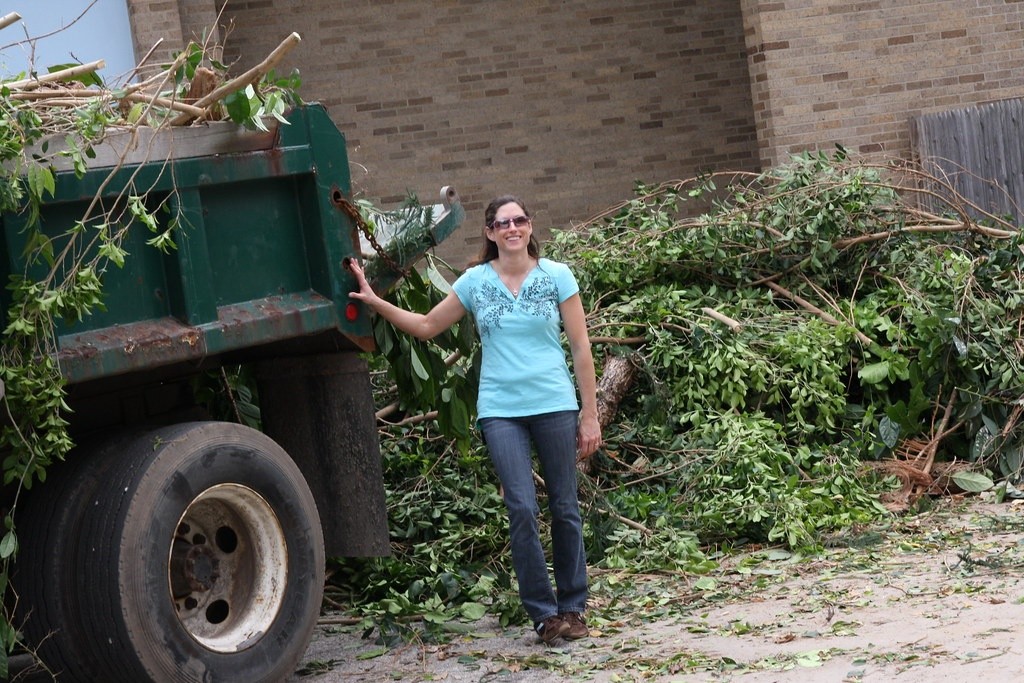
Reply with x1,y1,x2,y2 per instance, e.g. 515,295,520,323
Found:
535,616,570,642
560,613,590,638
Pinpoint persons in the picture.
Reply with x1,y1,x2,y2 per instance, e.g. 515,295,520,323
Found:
349,195,602,643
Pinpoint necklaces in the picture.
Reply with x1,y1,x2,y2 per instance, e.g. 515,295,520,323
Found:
494,260,533,296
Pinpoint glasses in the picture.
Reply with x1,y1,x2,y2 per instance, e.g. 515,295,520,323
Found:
490,215,531,229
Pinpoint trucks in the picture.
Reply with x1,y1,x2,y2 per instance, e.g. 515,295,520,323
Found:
0,100,464,683
